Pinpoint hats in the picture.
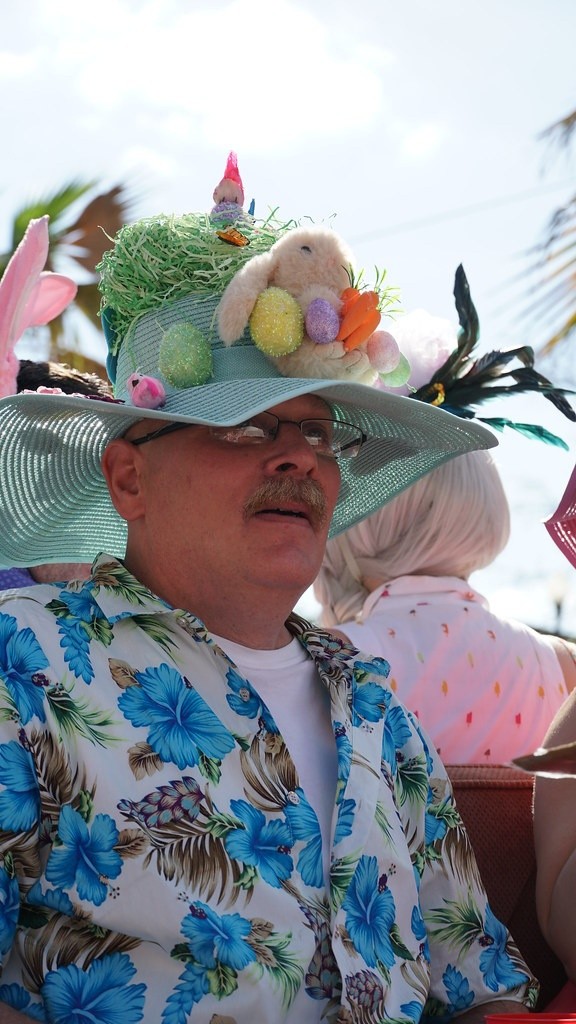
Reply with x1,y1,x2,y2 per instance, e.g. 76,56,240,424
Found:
0,152,576,568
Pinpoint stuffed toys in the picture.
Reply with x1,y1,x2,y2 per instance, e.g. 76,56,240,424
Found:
217,228,415,388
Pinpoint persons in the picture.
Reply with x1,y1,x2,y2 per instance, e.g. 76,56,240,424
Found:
0,150,537,1023
313,264,576,766
0,359,120,589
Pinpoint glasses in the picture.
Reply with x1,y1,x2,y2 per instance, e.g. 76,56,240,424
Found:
132,411,367,460
